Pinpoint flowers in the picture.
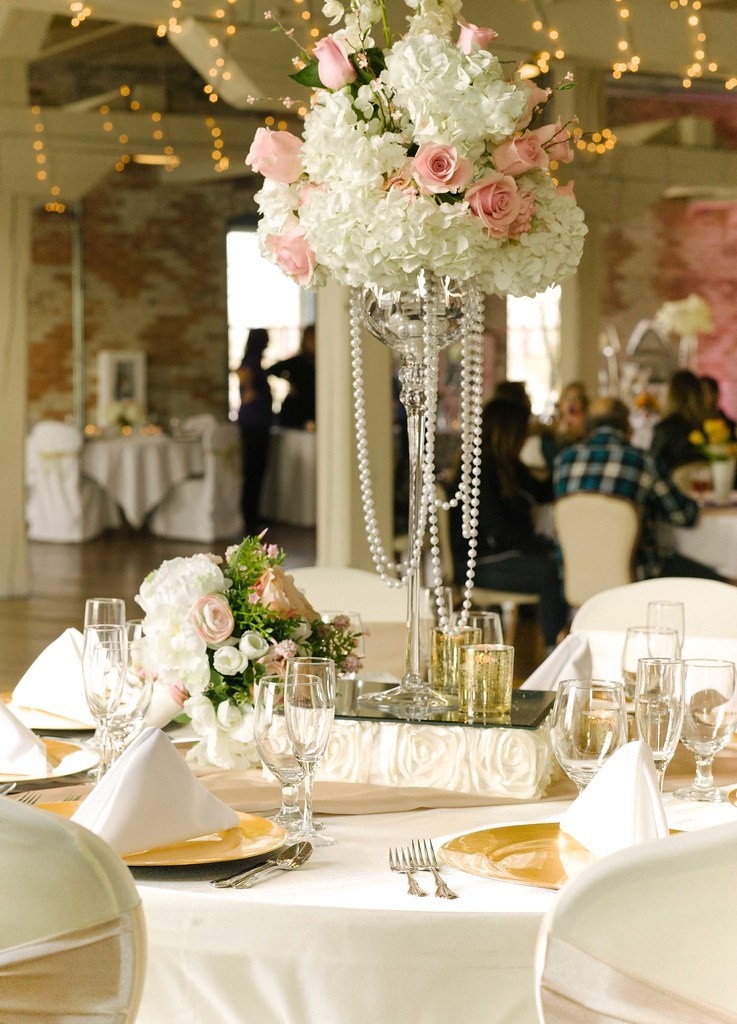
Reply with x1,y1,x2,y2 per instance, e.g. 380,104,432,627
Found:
656,293,717,336
636,388,660,419
245,0,589,300
690,418,737,461
135,528,371,772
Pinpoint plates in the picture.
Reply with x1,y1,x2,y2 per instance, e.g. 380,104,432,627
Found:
32,803,286,866
438,822,688,890
0,739,102,782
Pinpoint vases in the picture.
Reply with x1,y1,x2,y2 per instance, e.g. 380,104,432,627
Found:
712,461,737,505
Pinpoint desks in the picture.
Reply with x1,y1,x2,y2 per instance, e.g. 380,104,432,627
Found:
82,434,189,528
0,739,737,1024
534,505,737,582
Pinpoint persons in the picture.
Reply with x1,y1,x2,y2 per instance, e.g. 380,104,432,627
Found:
392,369,737,657
236,324,316,546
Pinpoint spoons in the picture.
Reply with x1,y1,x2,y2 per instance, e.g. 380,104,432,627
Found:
230,841,313,888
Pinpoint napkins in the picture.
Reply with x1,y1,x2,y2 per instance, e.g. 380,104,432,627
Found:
12,626,93,724
559,741,670,855
520,625,592,706
69,726,241,857
0,700,49,776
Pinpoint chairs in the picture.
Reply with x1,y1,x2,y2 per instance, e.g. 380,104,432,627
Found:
283,566,432,685
23,413,243,544
432,486,540,649
531,823,737,1024
259,428,316,528
554,492,640,642
0,796,147,1023
572,576,737,713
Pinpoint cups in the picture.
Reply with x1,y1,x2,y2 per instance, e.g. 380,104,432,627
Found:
648,601,685,658
320,611,365,657
451,612,504,645
430,626,482,697
550,679,629,797
465,644,514,715
622,626,681,704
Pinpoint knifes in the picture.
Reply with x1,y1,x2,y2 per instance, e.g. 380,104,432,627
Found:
209,848,284,888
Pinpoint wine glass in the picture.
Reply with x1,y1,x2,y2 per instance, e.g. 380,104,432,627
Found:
284,657,336,847
634,659,685,797
253,674,327,835
82,598,153,787
688,466,711,508
672,659,737,804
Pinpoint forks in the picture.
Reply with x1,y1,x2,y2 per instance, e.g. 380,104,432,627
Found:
411,839,460,900
388,846,429,897
64,793,82,802
18,792,41,806
